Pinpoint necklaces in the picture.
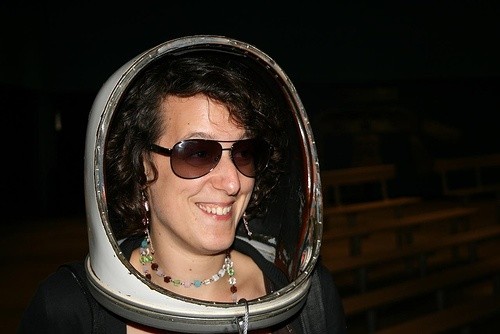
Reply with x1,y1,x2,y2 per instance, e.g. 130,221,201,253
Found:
139,239,238,295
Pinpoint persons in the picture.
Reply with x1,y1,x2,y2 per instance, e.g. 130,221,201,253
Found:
17,33,348,334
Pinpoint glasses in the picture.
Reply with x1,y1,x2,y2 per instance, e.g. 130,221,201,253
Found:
138,137,272,180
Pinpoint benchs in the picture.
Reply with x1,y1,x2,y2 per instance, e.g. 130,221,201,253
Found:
0,155,500,334
